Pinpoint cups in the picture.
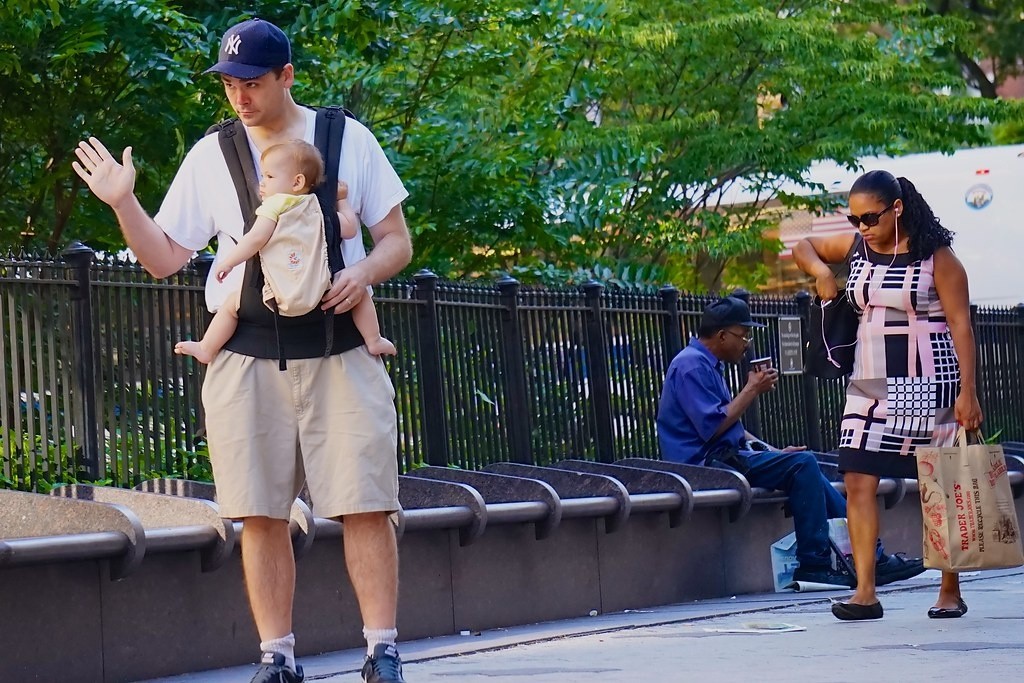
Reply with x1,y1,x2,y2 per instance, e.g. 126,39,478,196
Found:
750,357,774,372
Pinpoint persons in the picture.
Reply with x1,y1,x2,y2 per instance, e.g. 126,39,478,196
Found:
655,293,930,591
70,18,414,683
792,170,983,622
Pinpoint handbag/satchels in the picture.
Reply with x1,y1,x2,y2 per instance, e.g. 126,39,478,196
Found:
915,424,1024,571
805,235,860,378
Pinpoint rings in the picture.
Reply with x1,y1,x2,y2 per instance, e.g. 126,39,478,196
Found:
346,298,352,305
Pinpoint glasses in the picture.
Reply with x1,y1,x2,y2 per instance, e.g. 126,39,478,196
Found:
847,202,894,229
723,328,755,348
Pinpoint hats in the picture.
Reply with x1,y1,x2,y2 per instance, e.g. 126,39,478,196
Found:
201,18,291,79
702,297,768,328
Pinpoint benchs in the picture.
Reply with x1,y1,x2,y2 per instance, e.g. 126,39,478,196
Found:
0,443,1024,683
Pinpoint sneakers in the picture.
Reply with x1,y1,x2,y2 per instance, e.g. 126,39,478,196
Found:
792,562,858,588
873,551,929,587
360,641,405,683
250,652,305,682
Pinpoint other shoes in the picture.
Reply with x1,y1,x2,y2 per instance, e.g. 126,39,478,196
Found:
927,599,967,619
831,601,883,622
